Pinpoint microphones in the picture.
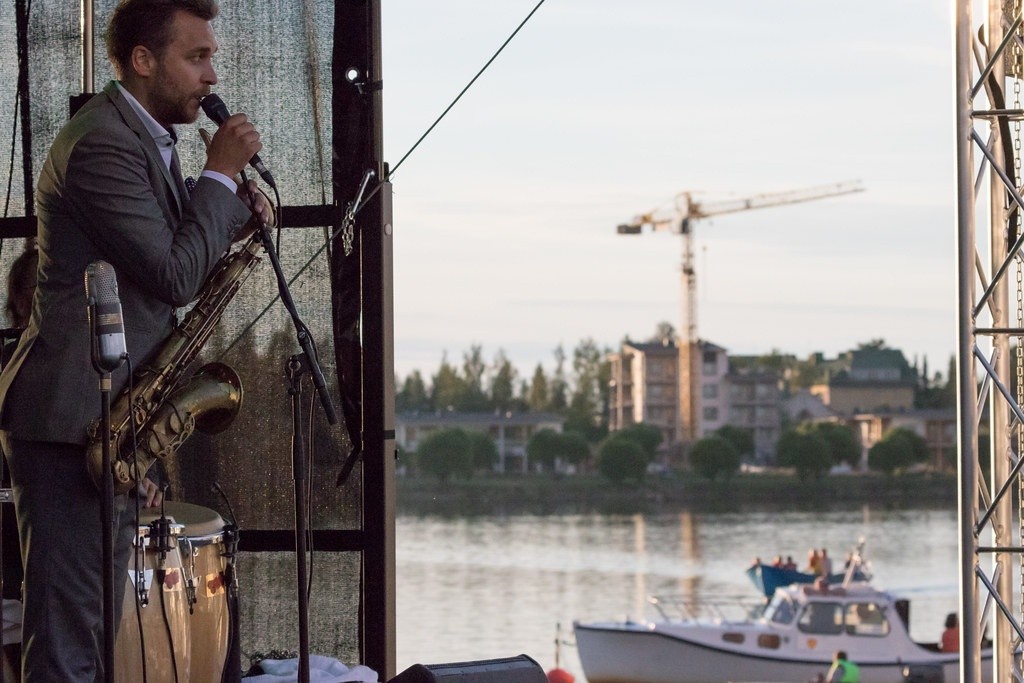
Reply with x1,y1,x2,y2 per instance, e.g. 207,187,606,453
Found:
84,261,127,373
200,93,275,189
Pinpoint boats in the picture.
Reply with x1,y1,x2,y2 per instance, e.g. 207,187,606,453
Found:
570,577,1023,683
746,564,872,596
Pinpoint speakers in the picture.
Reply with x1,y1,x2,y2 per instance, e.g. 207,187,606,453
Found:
385,654,549,683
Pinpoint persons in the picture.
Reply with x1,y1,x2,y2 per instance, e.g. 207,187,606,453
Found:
825,651,859,683
774,555,796,571
814,569,831,592
810,549,832,577
942,613,959,653
0,0,274,683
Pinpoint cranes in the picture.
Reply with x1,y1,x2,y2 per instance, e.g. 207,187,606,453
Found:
616,181,866,437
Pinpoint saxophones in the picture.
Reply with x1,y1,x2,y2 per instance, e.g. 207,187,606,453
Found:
88,129,268,497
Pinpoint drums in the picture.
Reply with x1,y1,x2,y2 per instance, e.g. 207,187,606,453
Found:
111,496,237,683
